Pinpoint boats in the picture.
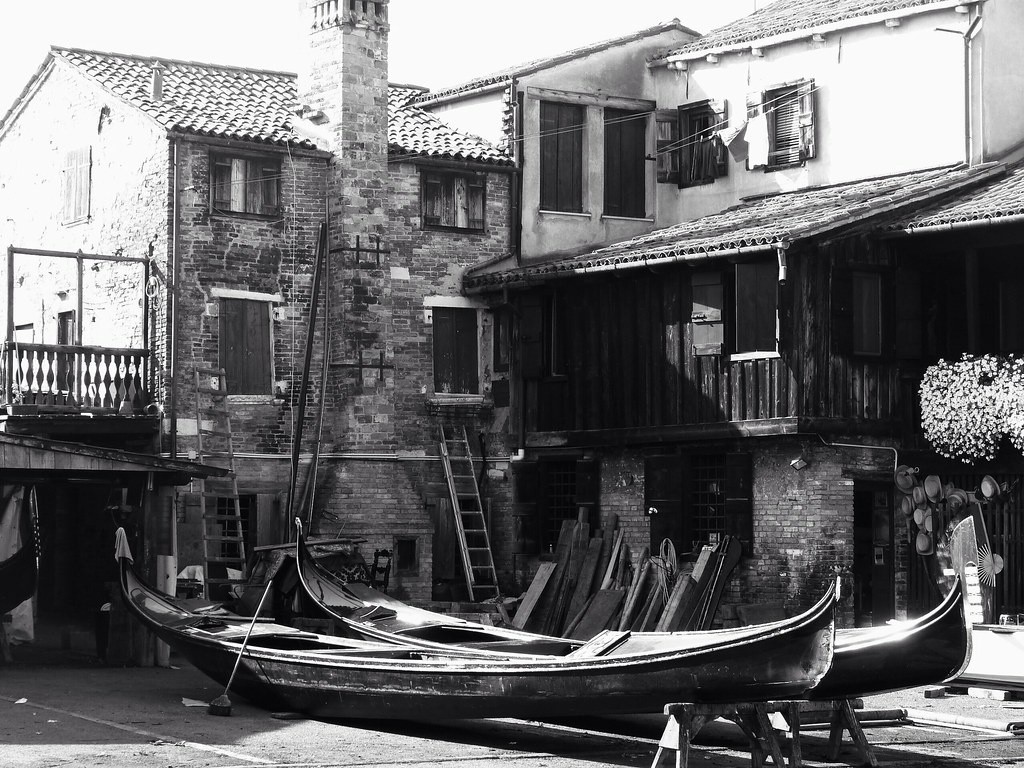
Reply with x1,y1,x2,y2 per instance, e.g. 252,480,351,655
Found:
118,516,966,727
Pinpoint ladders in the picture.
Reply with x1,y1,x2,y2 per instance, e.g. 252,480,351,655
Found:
438,422,501,601
191,365,250,603
